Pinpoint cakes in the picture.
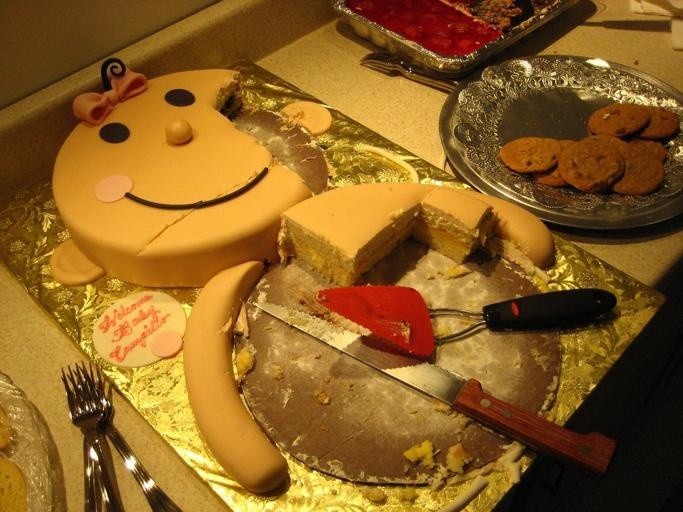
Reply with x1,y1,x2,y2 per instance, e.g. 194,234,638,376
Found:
48,56,555,492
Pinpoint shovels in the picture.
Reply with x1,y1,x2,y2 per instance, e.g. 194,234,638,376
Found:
316,286,617,358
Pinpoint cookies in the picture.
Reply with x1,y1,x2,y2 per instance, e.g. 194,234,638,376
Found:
501,104,680,198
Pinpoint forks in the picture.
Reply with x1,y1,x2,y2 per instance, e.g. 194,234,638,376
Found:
360,54,455,93
62,361,181,510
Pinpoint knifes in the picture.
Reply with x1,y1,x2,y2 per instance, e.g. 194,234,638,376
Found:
252,302,622,473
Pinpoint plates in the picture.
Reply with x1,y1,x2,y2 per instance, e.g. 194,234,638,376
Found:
439,54,683,231
